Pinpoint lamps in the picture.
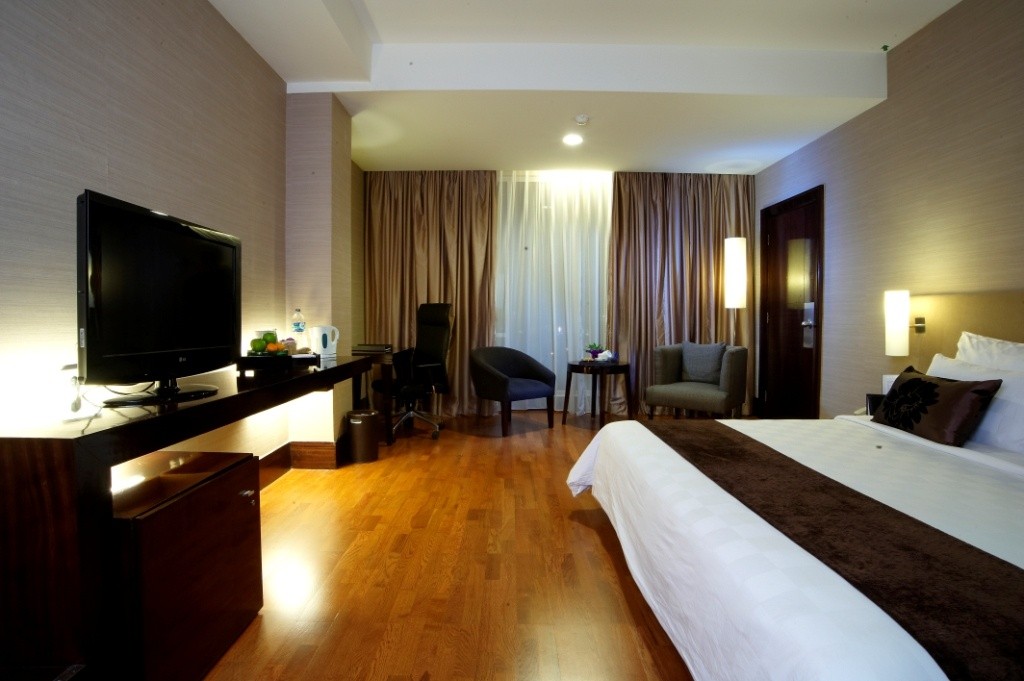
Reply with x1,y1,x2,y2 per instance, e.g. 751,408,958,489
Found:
884,291,926,357
723,237,748,309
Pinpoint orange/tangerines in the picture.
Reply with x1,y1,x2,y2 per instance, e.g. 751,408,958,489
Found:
266,343,285,350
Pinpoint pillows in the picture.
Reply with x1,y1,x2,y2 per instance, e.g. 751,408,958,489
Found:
923,353,1023,454
953,331,1024,372
870,365,1003,449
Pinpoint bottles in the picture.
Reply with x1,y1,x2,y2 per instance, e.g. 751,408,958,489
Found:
281,338,296,354
292,309,305,349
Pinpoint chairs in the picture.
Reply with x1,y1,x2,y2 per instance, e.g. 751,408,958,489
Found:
469,346,556,438
370,302,455,445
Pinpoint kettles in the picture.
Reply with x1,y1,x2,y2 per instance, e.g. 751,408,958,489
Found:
309,326,339,358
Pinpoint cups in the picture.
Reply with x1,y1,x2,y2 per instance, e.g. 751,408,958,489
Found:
256,331,276,339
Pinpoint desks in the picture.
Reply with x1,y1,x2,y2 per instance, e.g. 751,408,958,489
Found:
0,352,372,681
562,361,633,431
350,343,395,445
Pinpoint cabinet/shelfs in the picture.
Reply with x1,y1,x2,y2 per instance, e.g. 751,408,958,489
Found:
110,450,265,680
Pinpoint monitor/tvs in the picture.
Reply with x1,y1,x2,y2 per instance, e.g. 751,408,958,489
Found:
75,189,243,405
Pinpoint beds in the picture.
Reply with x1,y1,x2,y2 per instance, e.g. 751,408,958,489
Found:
566,290,1023,681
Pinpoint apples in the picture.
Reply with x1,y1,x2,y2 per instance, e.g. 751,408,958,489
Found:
251,333,277,351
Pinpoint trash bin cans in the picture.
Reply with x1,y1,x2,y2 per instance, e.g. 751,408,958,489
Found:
345,410,379,463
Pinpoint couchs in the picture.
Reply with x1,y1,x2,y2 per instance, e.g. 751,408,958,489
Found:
644,342,749,420
